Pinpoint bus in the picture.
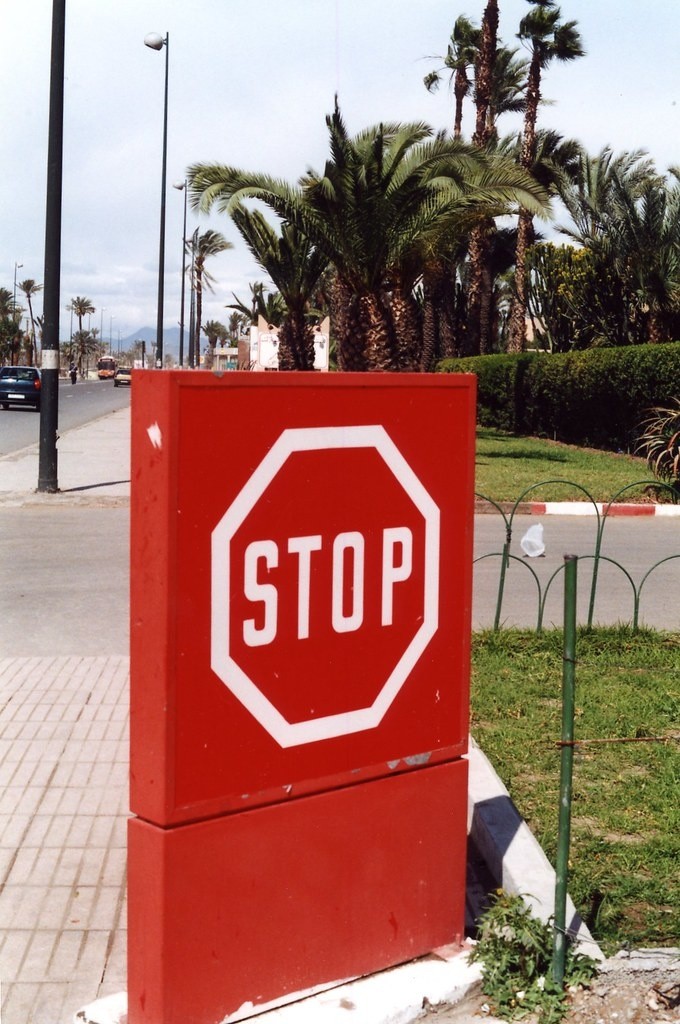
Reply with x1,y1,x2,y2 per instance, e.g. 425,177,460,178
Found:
98,356,118,380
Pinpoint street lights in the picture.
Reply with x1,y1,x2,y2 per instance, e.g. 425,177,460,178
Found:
110,315,115,356
100,308,107,343
12,261,23,322
143,30,170,372
173,180,188,368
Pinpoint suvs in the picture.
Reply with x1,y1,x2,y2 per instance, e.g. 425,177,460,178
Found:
114,367,132,387
0,366,41,411
